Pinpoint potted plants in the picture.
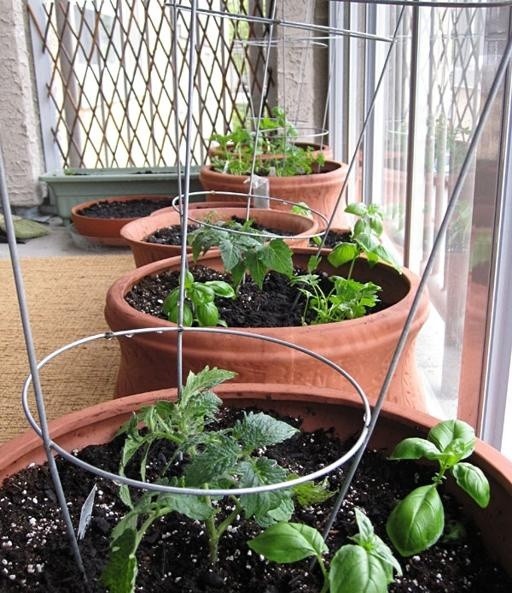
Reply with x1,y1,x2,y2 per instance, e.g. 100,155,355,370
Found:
0,365,511,590
119,206,319,268
208,137,330,158
357,106,499,323
106,202,430,408
199,105,349,228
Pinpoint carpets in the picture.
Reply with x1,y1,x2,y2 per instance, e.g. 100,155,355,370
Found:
0,250,142,451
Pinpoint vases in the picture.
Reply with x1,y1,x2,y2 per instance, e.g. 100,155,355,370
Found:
38,166,201,226
70,193,175,248
